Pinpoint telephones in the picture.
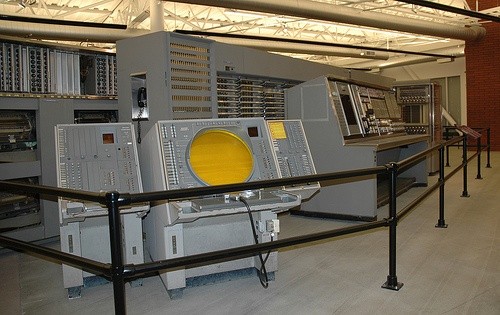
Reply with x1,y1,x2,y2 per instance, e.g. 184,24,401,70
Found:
137,86,148,108
